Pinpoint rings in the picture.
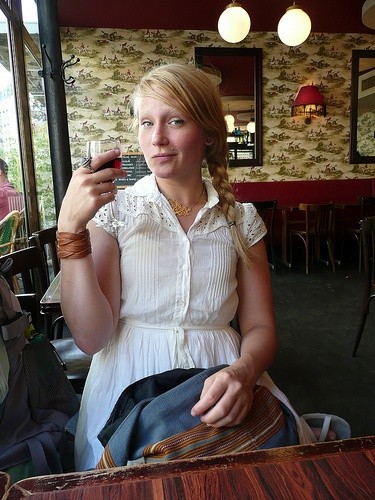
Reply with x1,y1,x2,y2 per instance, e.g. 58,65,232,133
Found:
80,155,95,173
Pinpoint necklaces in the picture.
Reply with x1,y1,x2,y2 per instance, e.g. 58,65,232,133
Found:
161,183,207,216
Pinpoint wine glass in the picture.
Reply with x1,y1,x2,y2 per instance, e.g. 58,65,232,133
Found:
88,140,128,227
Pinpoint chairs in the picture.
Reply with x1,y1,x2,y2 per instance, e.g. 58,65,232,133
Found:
0,208,93,395
237,196,375,357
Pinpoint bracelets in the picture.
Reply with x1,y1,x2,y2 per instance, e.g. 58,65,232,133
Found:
56,232,92,259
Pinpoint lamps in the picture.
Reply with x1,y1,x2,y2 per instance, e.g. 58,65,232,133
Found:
218,0,251,44
277,0,312,46
224,104,255,133
291,85,327,125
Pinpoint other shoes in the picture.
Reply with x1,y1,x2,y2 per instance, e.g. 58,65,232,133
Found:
302,414,350,443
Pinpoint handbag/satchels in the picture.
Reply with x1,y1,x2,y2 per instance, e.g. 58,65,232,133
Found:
1,342,81,474
90,362,295,471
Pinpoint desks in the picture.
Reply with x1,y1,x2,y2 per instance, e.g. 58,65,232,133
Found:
335,203,375,259
0,436,375,500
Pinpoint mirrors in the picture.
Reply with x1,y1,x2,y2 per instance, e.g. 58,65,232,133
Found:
193,47,264,167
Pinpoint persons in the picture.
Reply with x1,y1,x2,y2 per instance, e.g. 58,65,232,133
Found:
0,158,24,221
56,63,352,472
